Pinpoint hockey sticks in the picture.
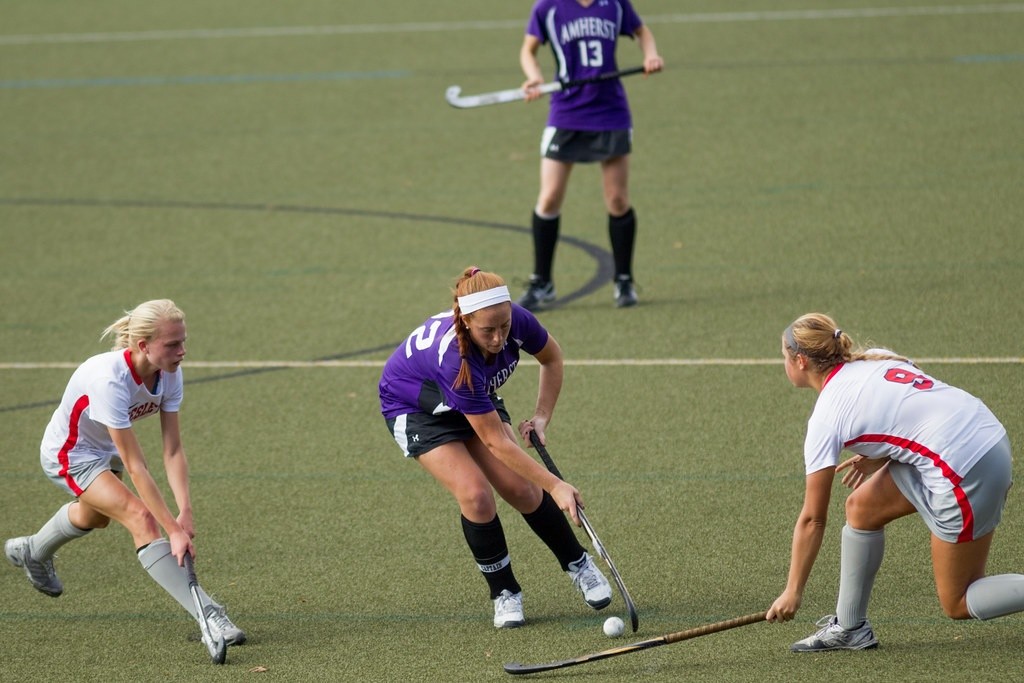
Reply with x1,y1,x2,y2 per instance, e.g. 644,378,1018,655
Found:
183,549,228,665
444,65,648,111
501,611,780,677
522,418,641,634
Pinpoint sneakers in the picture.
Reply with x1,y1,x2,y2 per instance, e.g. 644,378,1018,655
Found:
788,618,880,653
515,278,555,311
200,604,247,646
565,552,614,612
492,588,526,629
3,535,63,598
614,275,639,309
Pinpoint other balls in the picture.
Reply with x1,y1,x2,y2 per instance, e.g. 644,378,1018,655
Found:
601,615,626,639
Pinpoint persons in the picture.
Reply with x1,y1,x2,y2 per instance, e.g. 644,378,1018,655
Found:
515,0,664,313
4,299,246,648
378,267,613,628
765,312,1024,652
